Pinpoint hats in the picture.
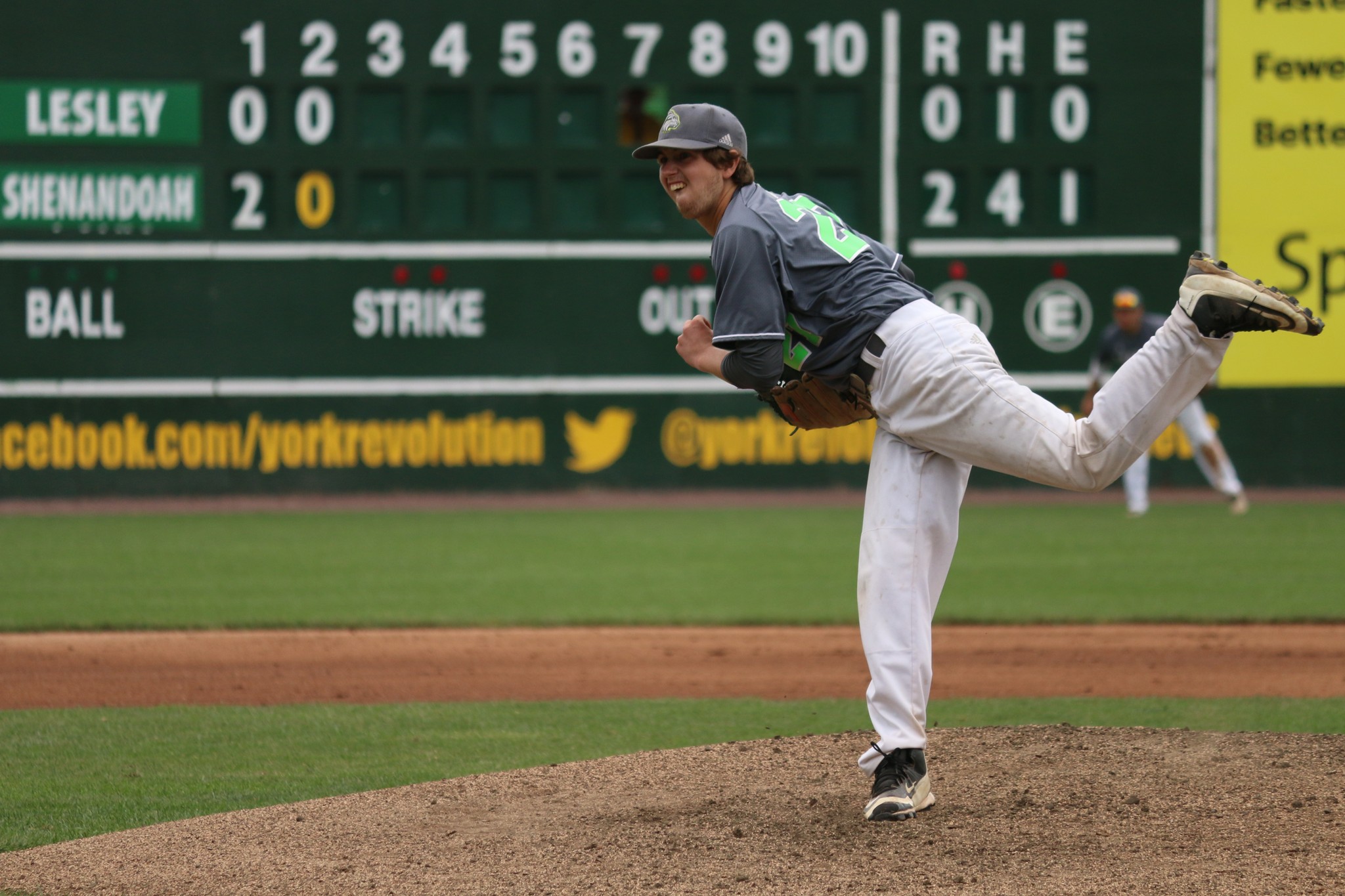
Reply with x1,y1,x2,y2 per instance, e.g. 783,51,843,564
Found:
632,103,748,158
1113,287,1143,309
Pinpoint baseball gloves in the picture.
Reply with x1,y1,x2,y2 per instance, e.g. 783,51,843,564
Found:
769,371,881,432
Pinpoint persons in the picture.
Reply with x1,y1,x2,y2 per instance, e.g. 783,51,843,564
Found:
631,104,1326,822
1080,288,1249,515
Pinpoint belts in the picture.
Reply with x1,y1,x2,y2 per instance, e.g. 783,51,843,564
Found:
849,333,885,384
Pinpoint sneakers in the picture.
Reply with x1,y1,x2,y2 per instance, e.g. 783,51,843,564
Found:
865,740,936,821
1178,250,1325,338
